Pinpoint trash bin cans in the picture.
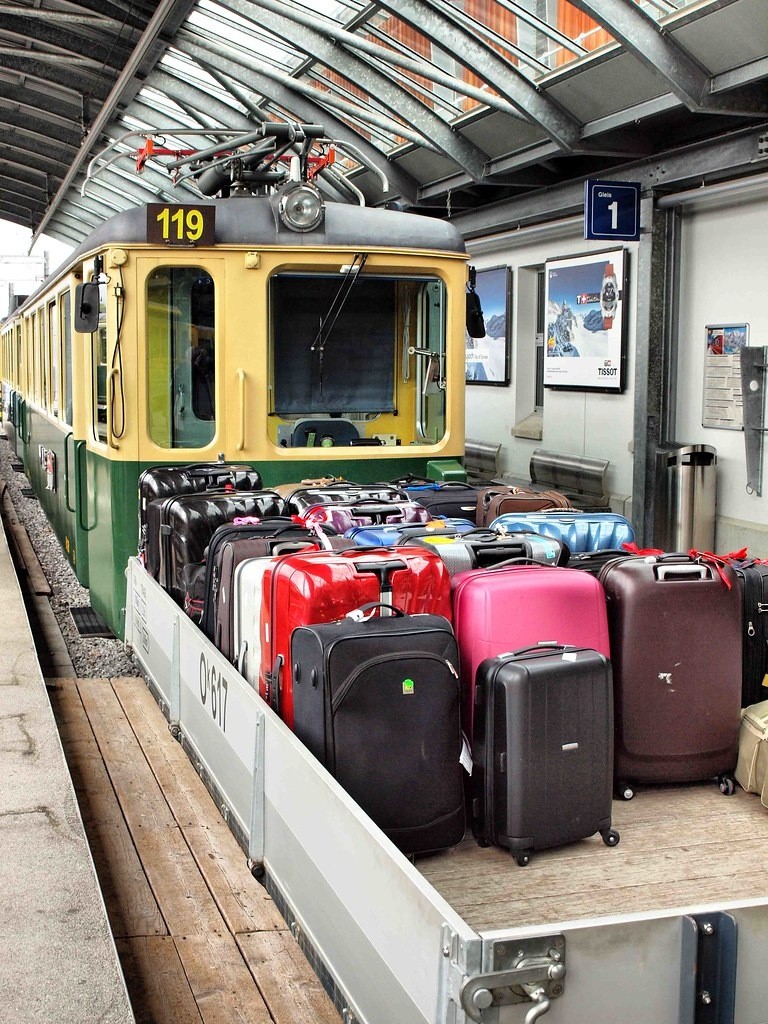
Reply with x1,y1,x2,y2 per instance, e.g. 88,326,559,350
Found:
655,441,717,555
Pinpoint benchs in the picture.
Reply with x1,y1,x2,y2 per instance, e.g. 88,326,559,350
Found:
490,447,615,499
455,435,502,481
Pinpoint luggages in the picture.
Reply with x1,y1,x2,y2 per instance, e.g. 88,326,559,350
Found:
135,461,767,866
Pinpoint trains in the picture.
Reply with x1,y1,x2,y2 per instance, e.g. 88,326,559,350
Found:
0,131,468,643
